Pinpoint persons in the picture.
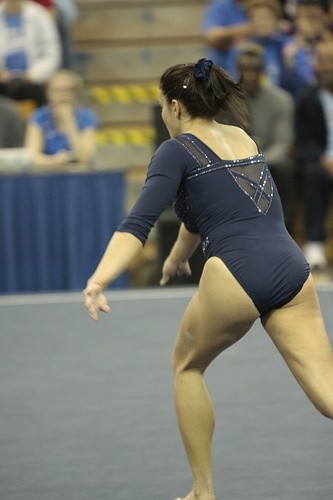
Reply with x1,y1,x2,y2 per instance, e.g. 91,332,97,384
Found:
83,58,333,500
0,0,101,172
200,0,333,267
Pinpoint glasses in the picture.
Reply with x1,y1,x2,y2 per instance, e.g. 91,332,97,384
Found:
242,63,263,73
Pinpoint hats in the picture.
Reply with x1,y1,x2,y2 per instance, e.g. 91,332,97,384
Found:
238,42,267,59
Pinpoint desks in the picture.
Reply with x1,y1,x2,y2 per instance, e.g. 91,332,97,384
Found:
0,168,130,292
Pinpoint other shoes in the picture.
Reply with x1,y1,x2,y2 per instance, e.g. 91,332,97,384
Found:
302,240,327,271
